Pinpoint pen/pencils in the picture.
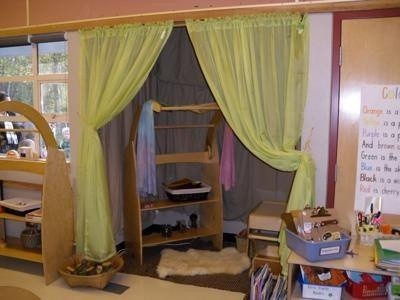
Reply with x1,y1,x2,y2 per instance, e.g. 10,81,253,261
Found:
358,204,383,230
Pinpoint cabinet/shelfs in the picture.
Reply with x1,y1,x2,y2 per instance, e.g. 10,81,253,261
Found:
123,101,225,267
247,201,287,277
0,98,74,286
286,238,400,299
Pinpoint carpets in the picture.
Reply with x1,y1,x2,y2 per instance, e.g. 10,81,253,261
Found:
155,246,252,280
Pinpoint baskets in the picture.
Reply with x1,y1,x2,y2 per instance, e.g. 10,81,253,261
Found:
344,273,392,299
57,253,125,289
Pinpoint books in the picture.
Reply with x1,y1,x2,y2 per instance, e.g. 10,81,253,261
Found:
373,234,400,300
248,262,285,299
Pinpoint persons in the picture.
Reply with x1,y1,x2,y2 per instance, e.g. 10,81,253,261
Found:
0,91,27,155
59,126,70,155
